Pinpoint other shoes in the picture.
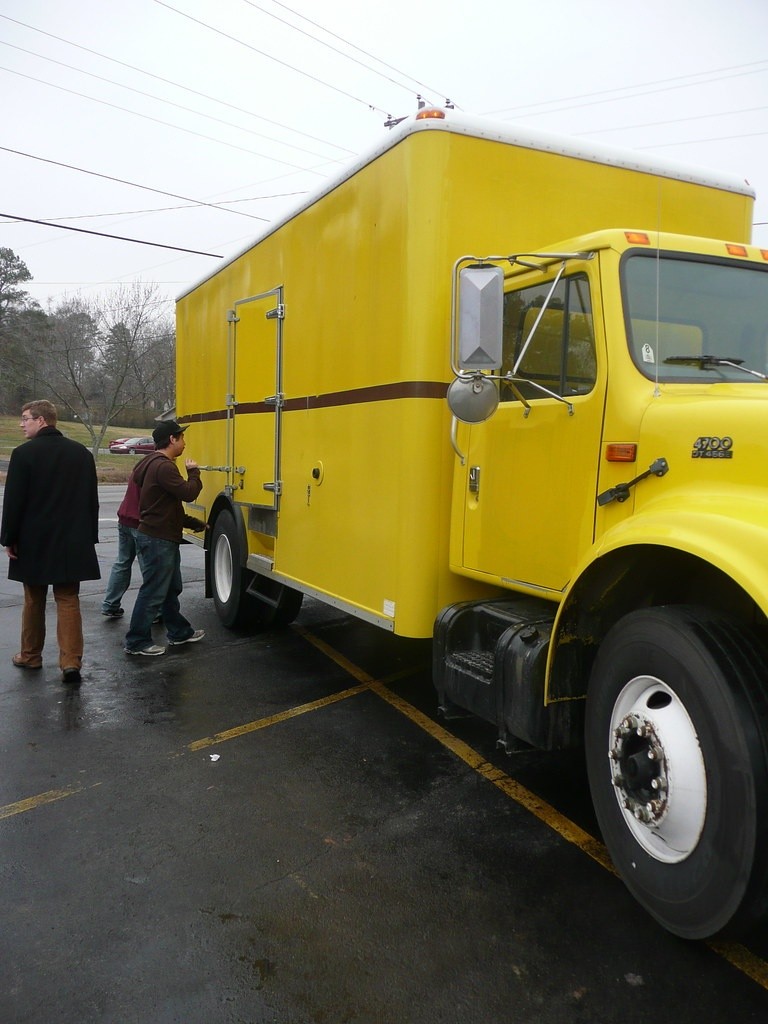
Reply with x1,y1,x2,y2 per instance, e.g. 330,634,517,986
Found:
11,651,43,668
62,667,81,682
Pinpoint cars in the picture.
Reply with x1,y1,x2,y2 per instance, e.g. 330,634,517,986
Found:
108,435,155,454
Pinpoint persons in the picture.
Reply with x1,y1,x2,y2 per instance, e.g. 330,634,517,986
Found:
102,441,177,624
123,420,210,656
0,399,100,689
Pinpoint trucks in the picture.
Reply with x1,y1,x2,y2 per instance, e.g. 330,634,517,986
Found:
174,106,767,942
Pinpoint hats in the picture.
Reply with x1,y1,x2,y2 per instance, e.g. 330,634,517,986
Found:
152,419,190,446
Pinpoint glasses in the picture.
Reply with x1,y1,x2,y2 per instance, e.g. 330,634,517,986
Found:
22,415,38,422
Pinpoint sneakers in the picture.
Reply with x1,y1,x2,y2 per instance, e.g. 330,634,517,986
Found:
101,607,124,618
153,617,160,624
169,629,206,645
123,644,166,656
